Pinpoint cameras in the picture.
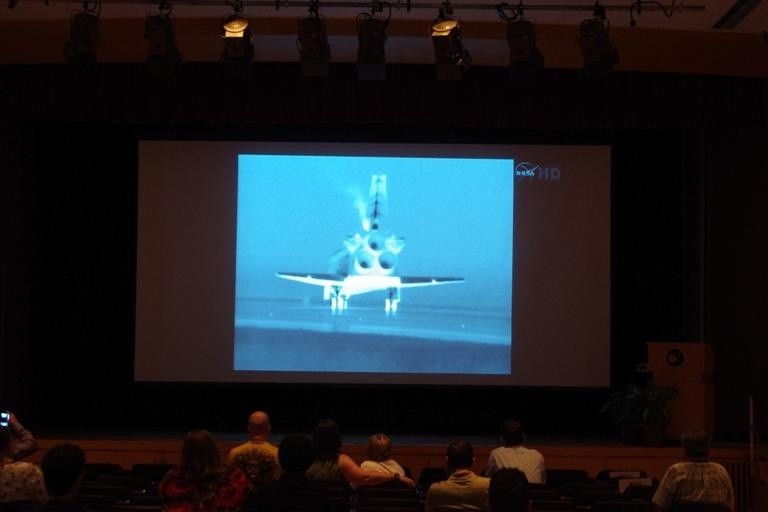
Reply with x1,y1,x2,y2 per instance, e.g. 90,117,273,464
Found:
0,412,10,429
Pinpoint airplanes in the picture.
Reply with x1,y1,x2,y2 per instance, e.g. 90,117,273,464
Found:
271,171,469,317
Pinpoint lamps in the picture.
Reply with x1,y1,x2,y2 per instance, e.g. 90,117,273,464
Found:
68,1,620,90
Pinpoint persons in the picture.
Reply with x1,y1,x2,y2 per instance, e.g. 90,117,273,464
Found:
0,411,88,512
652,425,735,512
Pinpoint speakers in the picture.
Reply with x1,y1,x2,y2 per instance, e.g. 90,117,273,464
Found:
647,342,716,443
